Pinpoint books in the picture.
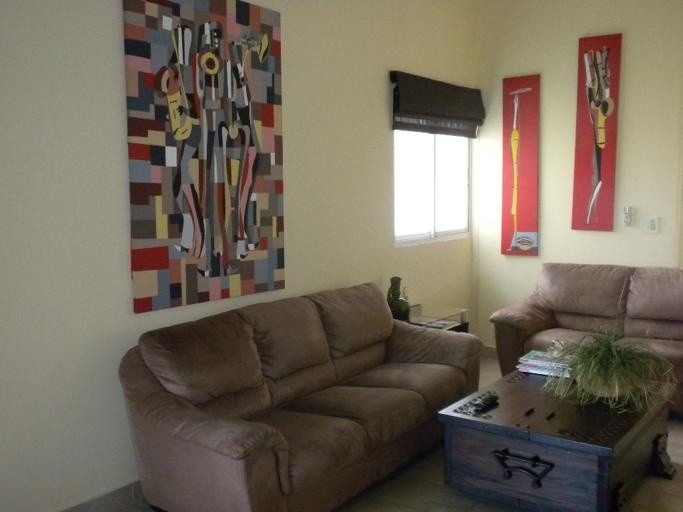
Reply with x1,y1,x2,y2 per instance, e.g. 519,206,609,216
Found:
514,348,577,379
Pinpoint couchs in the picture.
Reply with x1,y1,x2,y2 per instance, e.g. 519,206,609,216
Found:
489,261,683,414
117,282,485,511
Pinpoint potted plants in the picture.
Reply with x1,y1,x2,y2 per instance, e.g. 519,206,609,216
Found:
540,329,676,412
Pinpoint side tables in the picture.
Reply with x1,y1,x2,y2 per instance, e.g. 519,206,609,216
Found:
409,302,470,334
